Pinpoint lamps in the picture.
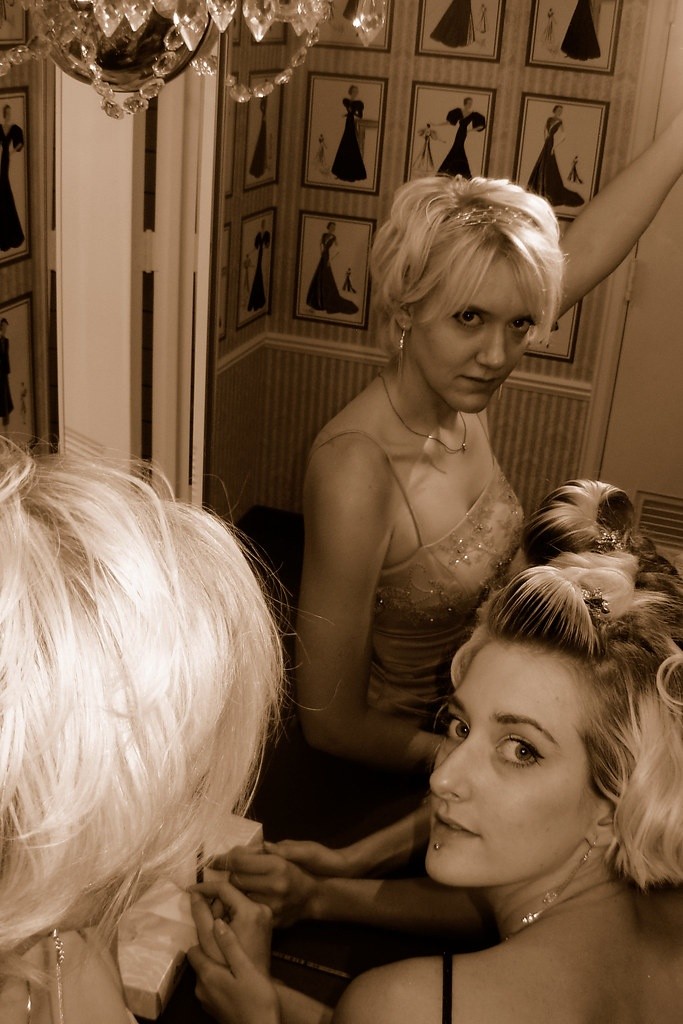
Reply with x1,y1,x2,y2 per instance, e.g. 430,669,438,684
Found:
0,0,389,118
414,0,506,64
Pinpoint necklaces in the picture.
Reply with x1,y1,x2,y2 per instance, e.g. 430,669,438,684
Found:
381,376,467,451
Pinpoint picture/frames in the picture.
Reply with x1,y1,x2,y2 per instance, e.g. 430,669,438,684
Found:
403,81,497,185
251,0,289,47
522,297,584,364
300,71,389,197
223,72,239,198
525,0,624,76
243,69,284,192
512,91,610,222
0,291,39,454
309,0,394,54
0,0,27,46
218,221,232,341
0,85,32,268
236,207,277,330
292,210,377,331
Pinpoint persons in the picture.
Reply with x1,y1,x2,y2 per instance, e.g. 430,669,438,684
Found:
296,113,683,770
205,477,683,931
185,549,683,1023
0,442,284,1023
